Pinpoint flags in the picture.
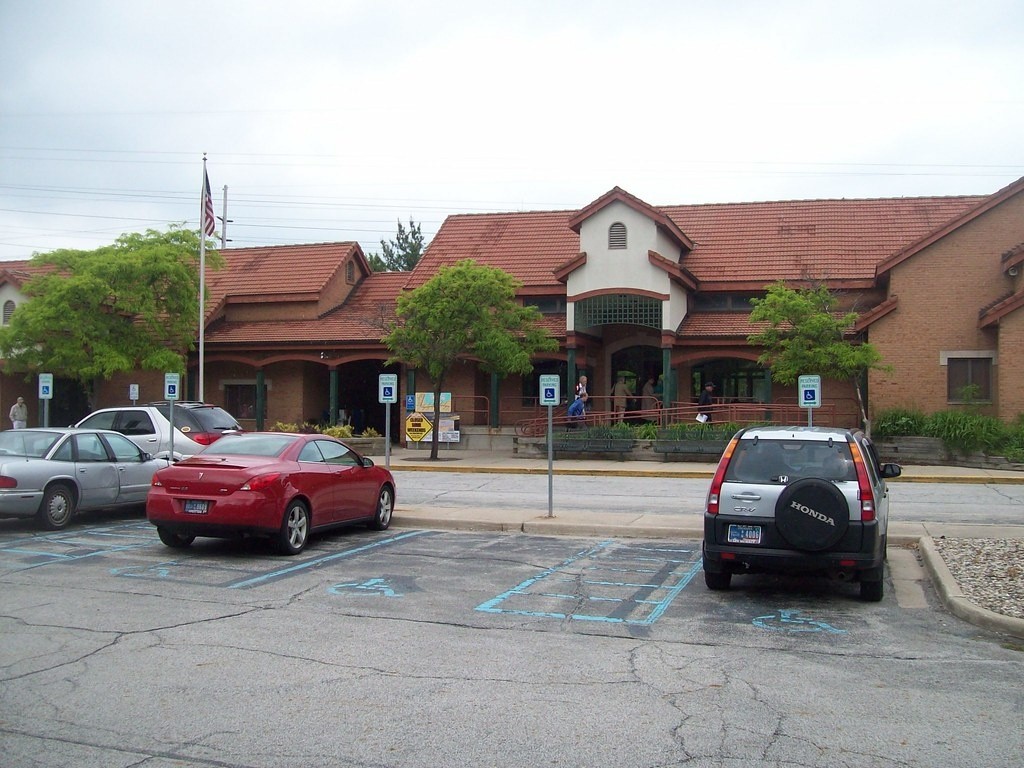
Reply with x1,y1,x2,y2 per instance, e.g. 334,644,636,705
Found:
200,167,215,238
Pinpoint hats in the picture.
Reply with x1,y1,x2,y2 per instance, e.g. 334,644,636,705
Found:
704,382,716,387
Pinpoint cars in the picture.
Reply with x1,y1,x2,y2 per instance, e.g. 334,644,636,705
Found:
0,427,176,530
146,431,397,554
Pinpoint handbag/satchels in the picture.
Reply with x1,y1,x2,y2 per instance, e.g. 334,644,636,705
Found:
610,383,617,399
564,415,577,428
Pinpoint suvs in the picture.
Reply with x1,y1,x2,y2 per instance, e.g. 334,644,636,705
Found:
68,401,243,462
702,426,900,602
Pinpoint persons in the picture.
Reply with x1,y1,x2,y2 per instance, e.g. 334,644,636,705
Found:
566,374,717,439
9,397,28,429
